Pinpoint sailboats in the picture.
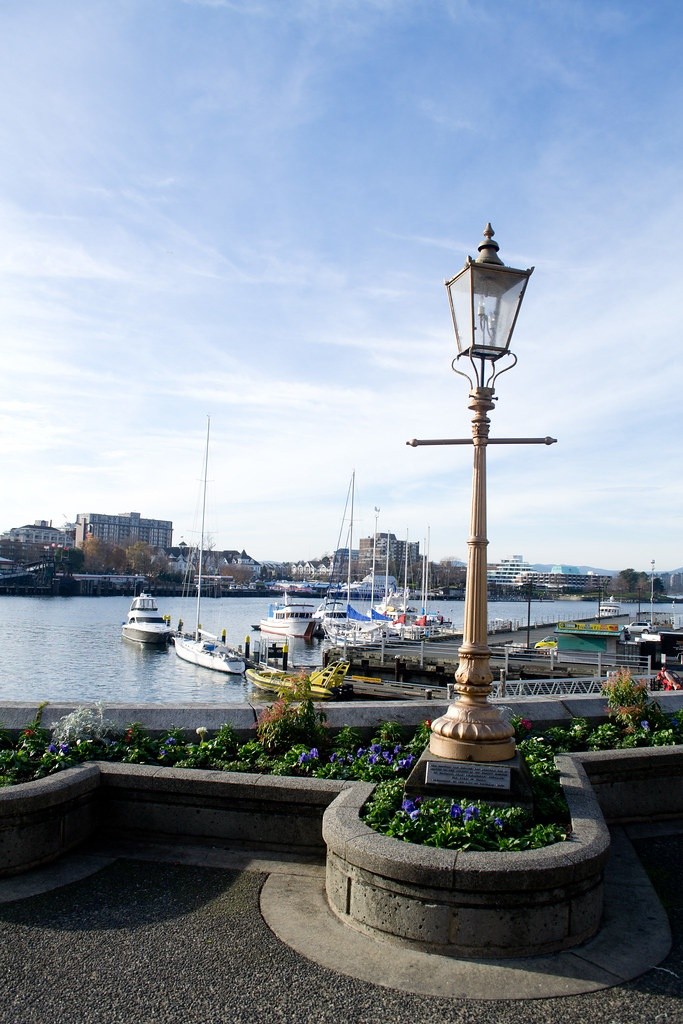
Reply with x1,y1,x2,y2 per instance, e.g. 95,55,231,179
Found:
171,415,245,674
318,469,453,648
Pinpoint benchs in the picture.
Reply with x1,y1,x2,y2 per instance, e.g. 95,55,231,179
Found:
488,640,514,647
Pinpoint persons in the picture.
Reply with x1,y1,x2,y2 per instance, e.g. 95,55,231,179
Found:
657,666,667,680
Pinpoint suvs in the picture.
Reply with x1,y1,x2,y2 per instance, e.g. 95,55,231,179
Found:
623,621,652,634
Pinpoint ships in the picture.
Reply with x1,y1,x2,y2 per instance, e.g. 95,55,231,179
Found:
327,574,405,600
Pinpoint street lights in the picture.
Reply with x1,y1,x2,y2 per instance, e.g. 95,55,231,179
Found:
404,224,558,801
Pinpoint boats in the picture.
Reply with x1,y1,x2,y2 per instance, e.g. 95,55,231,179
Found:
245,659,349,694
595,602,620,617
120,591,171,645
258,592,321,636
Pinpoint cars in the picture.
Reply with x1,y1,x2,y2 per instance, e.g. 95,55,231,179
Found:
534,636,559,648
